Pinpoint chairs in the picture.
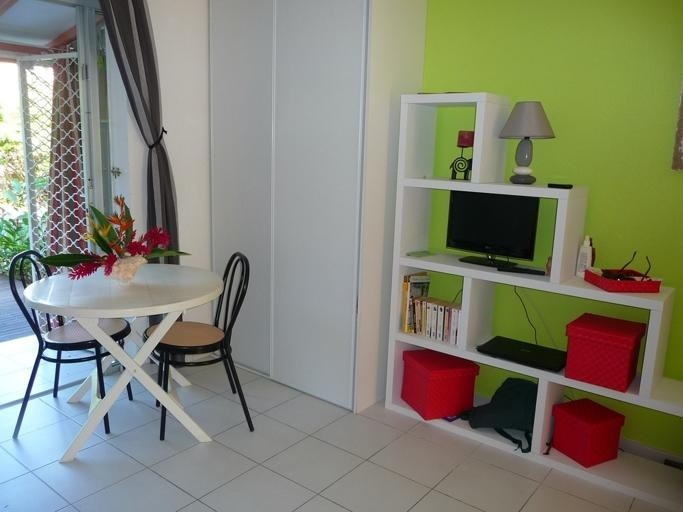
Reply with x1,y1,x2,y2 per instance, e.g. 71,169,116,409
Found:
7,249,135,442
142,249,256,441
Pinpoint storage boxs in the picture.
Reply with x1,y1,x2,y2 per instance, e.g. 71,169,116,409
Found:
400,345,479,421
551,313,647,469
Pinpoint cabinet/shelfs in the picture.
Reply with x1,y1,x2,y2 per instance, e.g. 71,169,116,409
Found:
383,89,681,512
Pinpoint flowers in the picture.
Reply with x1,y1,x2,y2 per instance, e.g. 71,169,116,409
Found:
35,193,192,282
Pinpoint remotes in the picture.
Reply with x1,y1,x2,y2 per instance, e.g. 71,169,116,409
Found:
498,265,545,275
548,183,572,189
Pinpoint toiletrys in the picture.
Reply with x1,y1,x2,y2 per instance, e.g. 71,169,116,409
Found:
575,235,593,278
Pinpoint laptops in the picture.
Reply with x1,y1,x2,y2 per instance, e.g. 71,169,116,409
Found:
477,336,567,372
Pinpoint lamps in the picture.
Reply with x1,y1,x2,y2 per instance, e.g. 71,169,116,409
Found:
497,98,554,183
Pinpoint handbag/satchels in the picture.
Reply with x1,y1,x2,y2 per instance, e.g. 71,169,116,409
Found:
465,377,537,454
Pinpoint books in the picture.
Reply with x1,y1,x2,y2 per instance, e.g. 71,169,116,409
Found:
401,272,463,347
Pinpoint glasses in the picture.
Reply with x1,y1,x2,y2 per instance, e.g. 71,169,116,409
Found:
614,251,653,284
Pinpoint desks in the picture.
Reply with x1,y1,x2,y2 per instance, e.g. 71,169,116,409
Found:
23,262,225,462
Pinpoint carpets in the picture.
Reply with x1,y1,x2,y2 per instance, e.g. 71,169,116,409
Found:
0,274,51,342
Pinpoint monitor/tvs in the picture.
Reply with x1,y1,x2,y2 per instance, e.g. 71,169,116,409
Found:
447,190,539,267
